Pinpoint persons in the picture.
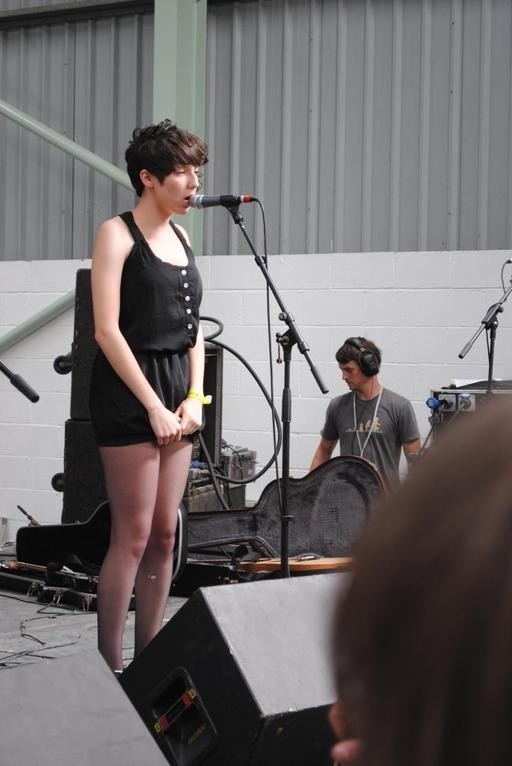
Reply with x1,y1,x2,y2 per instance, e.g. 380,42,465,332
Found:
326,391,512,766
89,118,208,678
310,336,421,495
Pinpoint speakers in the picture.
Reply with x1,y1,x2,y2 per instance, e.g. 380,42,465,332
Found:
0,650,170,766
118,568,357,766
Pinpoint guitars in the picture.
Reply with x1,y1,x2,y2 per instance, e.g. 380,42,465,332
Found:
8,561,99,593
194,550,358,573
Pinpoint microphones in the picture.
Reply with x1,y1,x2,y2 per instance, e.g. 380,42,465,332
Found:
189,193,253,209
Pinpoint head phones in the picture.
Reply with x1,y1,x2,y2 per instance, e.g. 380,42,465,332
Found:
345,336,379,376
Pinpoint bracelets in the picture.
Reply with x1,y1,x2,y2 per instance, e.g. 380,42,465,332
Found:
187,390,213,405
